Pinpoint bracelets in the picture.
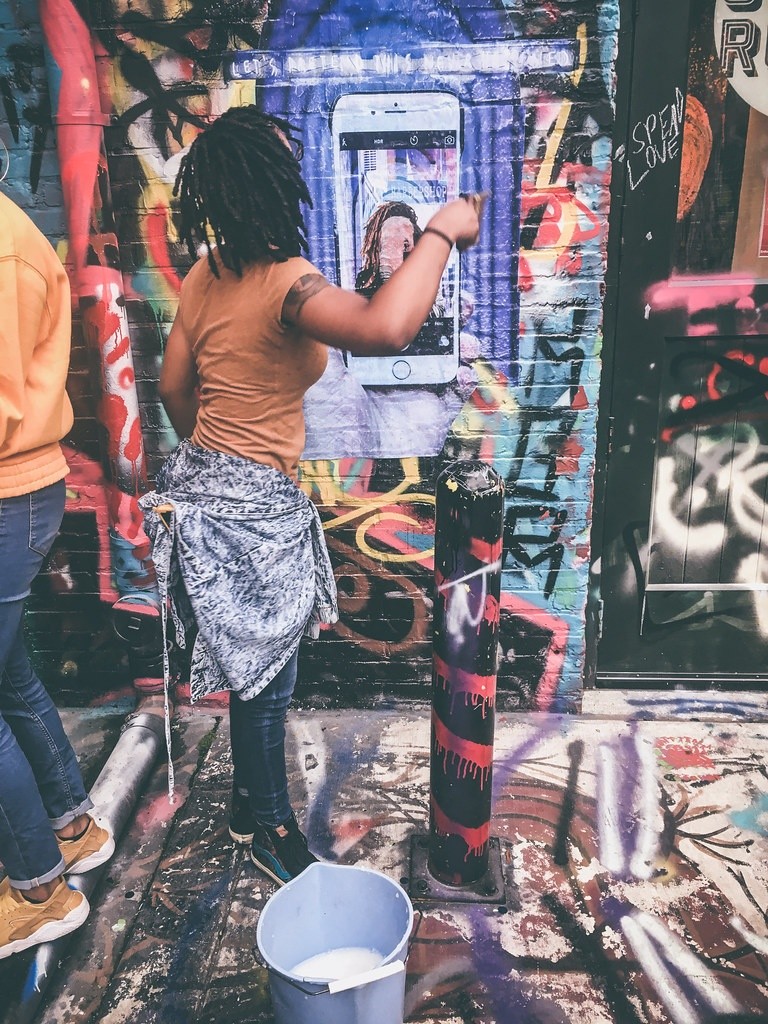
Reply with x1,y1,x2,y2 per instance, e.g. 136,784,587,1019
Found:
425,228,453,249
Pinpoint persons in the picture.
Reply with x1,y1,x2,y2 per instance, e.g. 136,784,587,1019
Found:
0,191,115,960
137,103,487,884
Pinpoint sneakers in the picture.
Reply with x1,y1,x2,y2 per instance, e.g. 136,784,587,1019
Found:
252,810,321,885
0,875,91,960
1,812,116,895
228,801,255,844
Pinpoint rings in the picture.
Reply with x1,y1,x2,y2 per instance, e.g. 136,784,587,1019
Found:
473,195,482,211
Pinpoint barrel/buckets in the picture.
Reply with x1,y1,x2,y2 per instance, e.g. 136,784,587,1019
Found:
252,861,423,1024
